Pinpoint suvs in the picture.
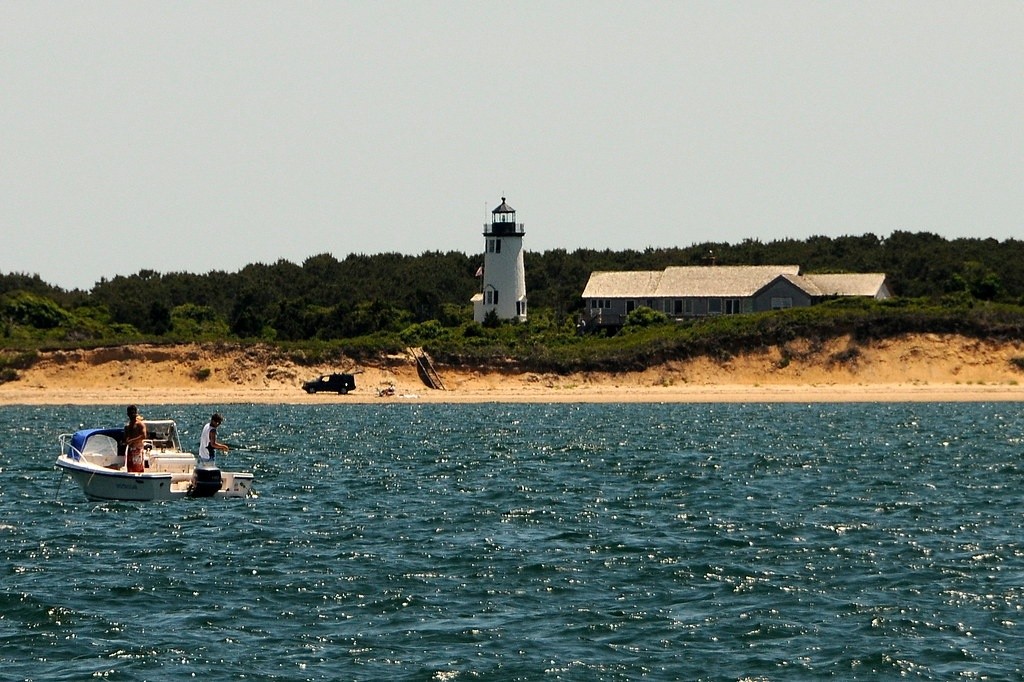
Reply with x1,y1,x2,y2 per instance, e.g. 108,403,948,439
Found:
302,373,356,395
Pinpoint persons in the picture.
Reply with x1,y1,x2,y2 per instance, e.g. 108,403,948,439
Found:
198,414,228,467
121,405,147,473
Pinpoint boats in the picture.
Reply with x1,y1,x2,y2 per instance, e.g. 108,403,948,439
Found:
53,419,255,504
